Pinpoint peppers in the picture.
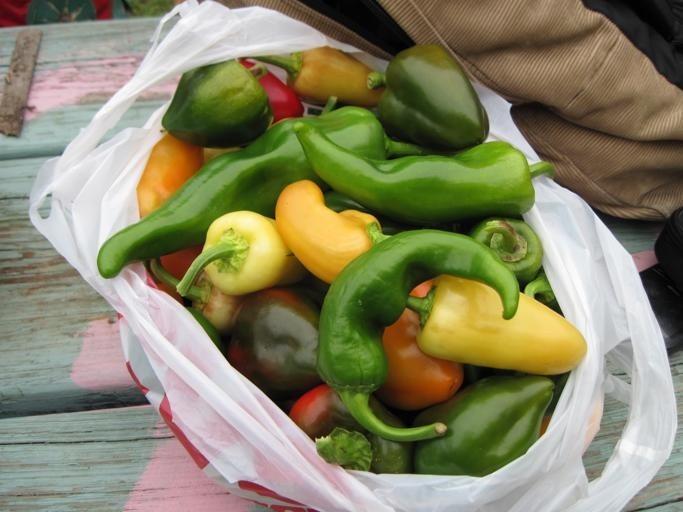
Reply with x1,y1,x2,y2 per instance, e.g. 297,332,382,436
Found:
96,43,586,477
